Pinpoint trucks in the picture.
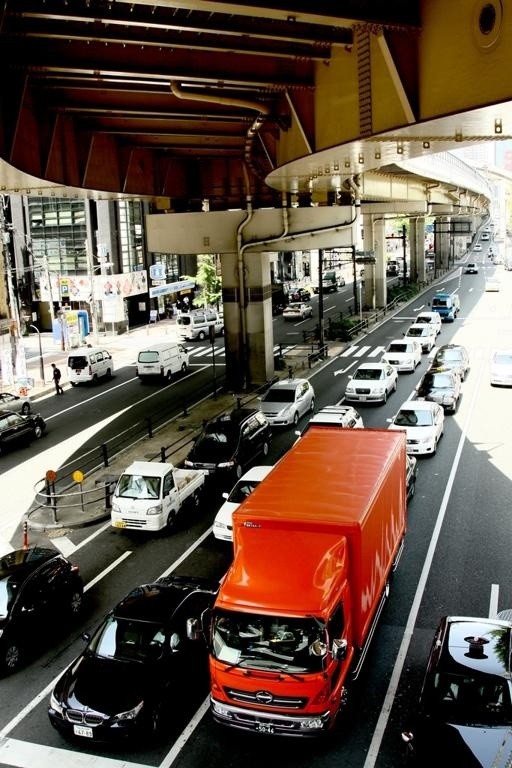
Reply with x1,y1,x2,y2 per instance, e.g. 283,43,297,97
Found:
205,420,414,743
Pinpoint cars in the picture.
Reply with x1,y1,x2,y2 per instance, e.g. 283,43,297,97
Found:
398,610,511,768
45,569,221,751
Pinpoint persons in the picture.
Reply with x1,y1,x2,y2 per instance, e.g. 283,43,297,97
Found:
217,618,261,639
49,363,63,395
397,412,417,423
207,426,228,442
135,477,157,496
150,627,179,653
163,297,192,319
104,273,145,294
